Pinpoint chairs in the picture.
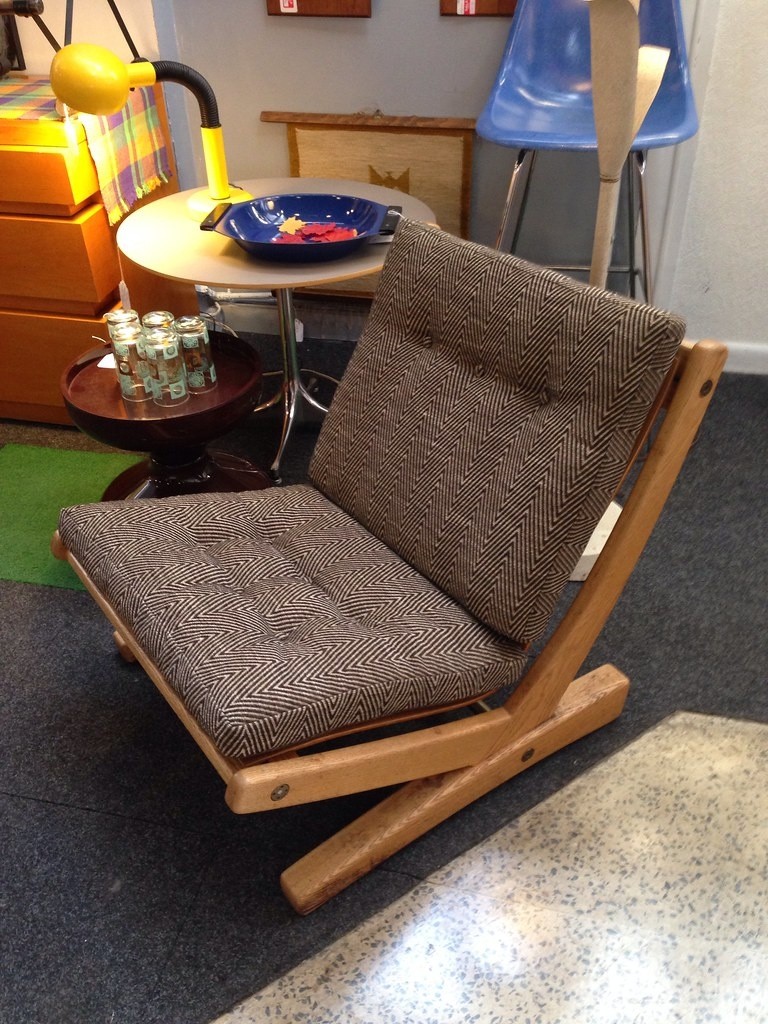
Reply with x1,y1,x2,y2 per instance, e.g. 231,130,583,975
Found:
53,220,730,914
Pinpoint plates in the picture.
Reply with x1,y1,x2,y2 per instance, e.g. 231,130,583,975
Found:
200,193,402,263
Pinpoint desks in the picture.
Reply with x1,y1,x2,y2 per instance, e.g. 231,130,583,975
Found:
116,178,438,479
61,329,269,500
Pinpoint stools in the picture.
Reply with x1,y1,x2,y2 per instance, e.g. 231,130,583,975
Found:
475,0,698,307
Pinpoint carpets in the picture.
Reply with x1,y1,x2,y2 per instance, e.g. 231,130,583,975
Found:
1,442,146,594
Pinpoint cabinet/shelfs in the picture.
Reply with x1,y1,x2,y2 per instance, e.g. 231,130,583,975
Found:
0,73,200,429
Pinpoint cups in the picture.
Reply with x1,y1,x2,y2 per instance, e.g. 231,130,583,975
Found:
141,311,186,378
173,315,218,394
143,326,190,408
110,322,155,402
106,309,141,348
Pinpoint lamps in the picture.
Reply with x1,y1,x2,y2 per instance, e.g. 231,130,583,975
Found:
47,46,259,215
